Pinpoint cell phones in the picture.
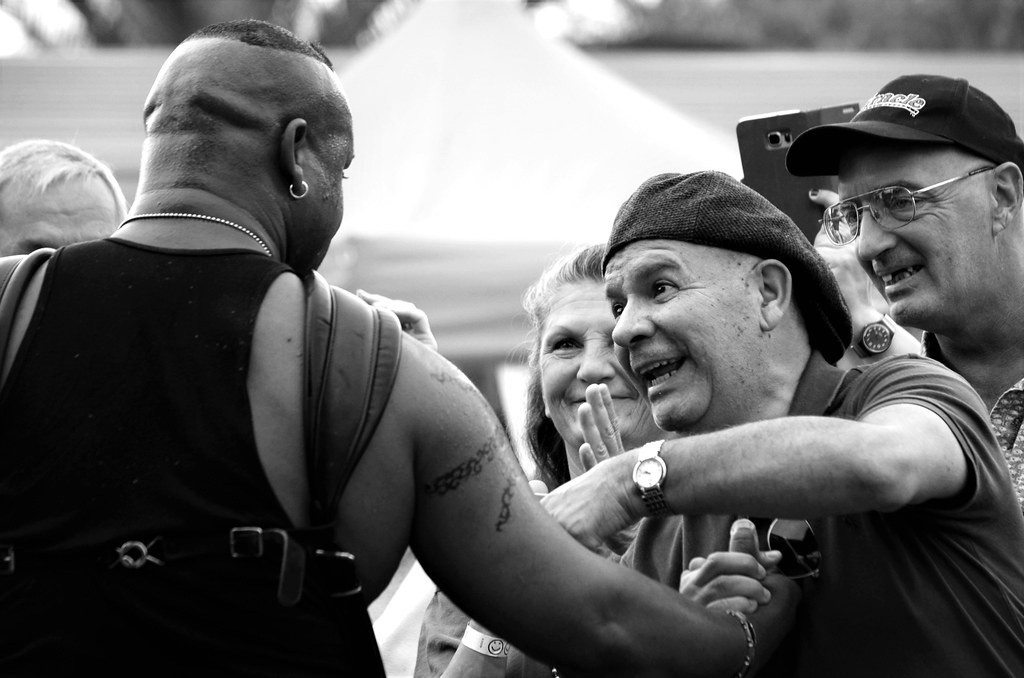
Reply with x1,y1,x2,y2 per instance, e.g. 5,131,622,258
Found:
736,103,861,246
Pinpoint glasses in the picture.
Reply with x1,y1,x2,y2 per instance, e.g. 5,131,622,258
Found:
817,164,1003,244
765,514,822,580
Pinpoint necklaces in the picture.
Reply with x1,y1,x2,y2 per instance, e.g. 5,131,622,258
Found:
119,212,274,258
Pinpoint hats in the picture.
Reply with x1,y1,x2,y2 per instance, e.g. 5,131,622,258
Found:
600,171,854,369
784,73,1024,181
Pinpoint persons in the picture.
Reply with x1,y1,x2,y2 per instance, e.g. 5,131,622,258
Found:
0,19,810,678
0,73,1024,678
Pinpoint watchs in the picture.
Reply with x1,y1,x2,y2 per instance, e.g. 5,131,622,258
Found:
632,438,676,520
851,313,896,359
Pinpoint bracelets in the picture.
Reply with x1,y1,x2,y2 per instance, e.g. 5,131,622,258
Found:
462,622,509,658
725,606,758,678
